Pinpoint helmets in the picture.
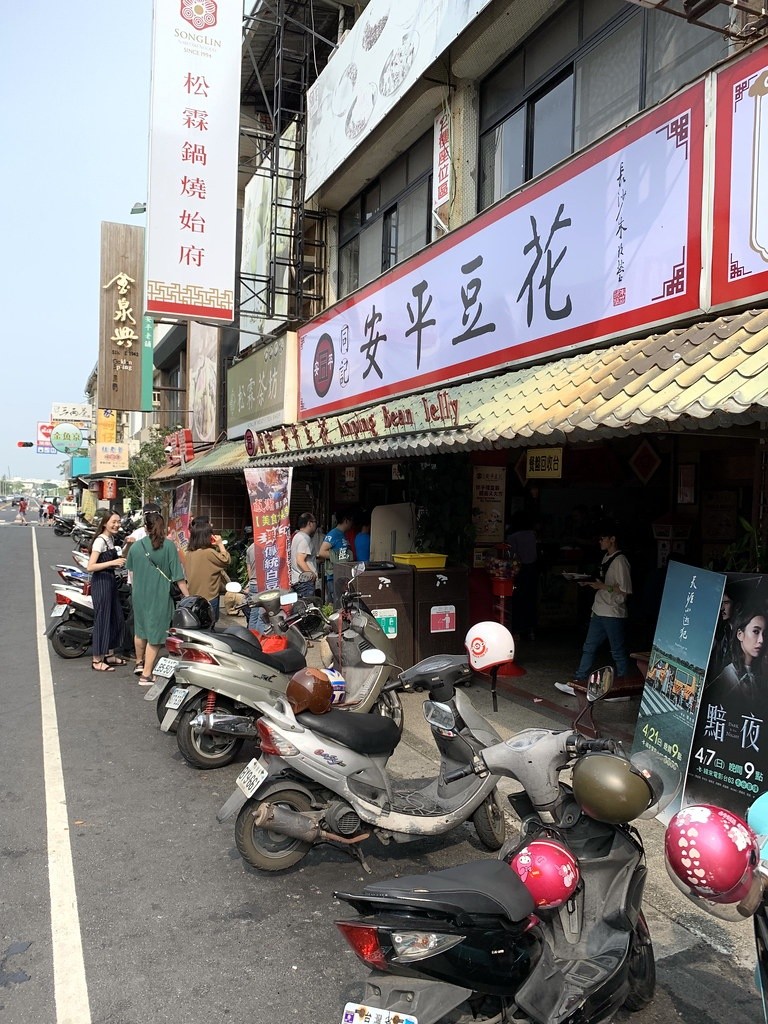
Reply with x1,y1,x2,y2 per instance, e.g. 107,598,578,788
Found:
510,839,579,910
286,666,335,716
665,805,759,904
571,752,664,824
322,668,346,704
177,593,216,631
291,596,323,636
465,620,516,672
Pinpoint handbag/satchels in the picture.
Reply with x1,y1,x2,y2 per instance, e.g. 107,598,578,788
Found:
83,581,92,595
16,513,21,520
252,626,287,654
169,579,189,609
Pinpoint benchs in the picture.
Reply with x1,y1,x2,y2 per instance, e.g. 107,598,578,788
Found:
567,678,643,738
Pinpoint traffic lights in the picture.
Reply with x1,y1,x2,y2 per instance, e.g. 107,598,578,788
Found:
18,442,34,448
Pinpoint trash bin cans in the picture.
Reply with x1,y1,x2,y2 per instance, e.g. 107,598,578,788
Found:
414,568,470,692
332,560,415,693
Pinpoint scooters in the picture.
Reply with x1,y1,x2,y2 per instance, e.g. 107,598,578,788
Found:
38,508,405,772
213,642,509,875
327,665,657,1023
732,788,768,1024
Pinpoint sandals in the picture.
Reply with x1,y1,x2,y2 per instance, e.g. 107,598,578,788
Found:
104,654,127,666
132,661,144,675
92,661,116,672
138,675,155,686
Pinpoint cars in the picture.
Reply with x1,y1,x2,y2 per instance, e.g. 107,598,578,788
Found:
44,495,59,505
0,494,30,507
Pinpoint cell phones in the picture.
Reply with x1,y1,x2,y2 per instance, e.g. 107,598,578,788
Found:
210,536,215,543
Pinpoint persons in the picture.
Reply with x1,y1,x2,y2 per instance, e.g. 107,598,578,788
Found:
184,515,231,621
18,498,28,526
554,531,633,702
38,502,55,526
318,510,370,597
126,515,190,685
87,511,127,671
707,584,768,702
122,504,162,585
291,513,318,596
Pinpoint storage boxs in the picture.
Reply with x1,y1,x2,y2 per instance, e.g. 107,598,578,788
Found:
391,553,449,568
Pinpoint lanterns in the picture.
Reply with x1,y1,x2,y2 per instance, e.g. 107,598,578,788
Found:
104,479,116,499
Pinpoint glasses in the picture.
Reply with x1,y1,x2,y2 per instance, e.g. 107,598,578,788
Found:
310,521,318,524
600,537,608,541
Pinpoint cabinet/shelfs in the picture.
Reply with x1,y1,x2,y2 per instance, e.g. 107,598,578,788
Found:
333,561,467,689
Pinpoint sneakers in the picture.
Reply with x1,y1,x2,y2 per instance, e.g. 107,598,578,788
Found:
553,680,576,697
603,691,631,702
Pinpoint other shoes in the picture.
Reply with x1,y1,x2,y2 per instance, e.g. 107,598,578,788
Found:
25,523,28,526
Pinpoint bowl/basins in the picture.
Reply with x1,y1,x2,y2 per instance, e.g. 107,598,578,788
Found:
563,572,591,579
